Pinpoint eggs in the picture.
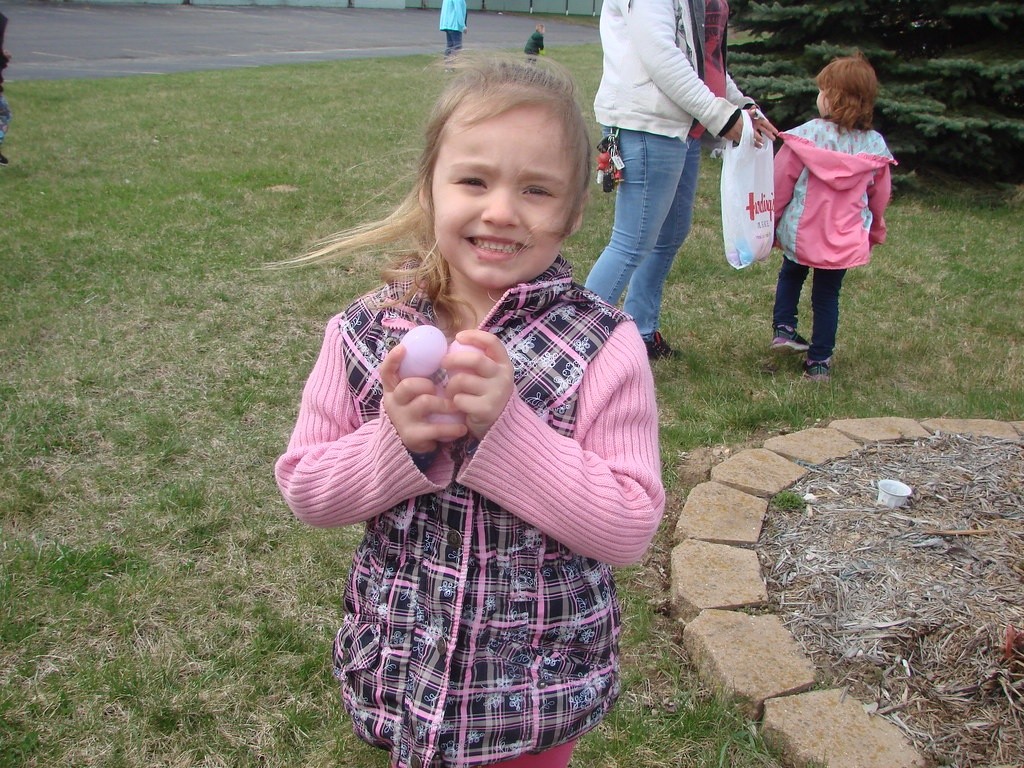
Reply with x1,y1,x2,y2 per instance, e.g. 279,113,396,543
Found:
448,340,484,378
398,325,447,378
427,385,466,443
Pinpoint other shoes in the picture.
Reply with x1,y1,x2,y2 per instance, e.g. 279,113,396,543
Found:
643,331,681,360
0,153,8,165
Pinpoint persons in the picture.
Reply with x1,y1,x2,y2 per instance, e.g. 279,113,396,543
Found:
593,0,778,360
439,0,469,72
0,12,12,166
772,50,898,382
524,24,545,61
273,51,666,768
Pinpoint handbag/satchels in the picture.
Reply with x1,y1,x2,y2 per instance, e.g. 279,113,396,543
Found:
720,109,775,270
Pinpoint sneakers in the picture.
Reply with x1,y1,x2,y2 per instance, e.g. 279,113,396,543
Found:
769,324,810,350
802,357,831,382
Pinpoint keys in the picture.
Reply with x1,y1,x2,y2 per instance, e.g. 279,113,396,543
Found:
596,134,626,194
